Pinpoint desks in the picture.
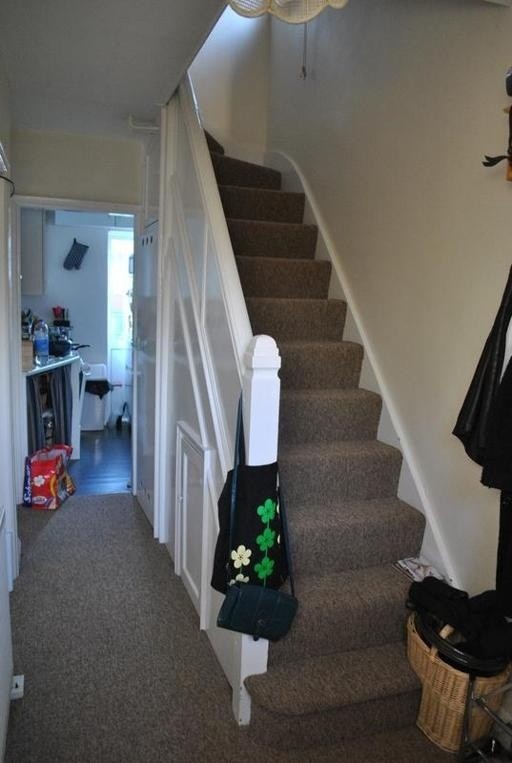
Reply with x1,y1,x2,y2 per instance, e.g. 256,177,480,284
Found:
22,354,81,460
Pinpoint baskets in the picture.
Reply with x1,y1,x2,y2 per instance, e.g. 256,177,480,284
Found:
407,610,512,756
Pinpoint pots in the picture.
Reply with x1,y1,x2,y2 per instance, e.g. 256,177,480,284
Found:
51,338,92,356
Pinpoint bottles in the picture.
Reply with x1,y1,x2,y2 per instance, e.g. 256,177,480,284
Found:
34,319,49,366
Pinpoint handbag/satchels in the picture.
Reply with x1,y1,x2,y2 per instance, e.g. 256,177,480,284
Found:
216,580,298,643
23,444,76,510
211,461,289,595
